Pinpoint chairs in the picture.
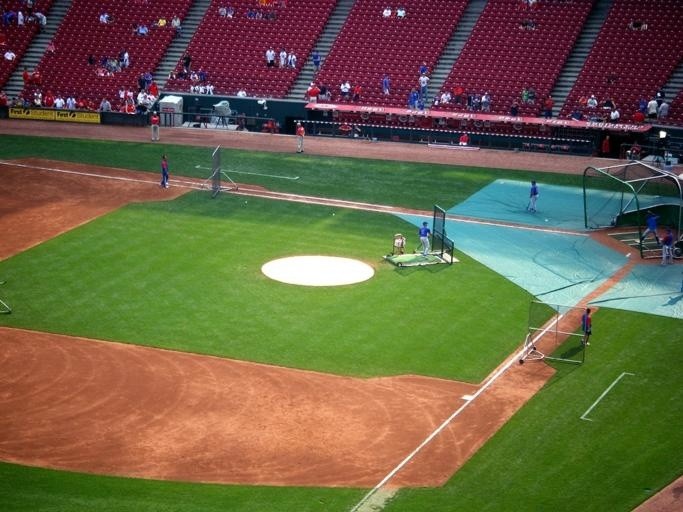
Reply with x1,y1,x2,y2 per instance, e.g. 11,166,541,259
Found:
0,0,683,135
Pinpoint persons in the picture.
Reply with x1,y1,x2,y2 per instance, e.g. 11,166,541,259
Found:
521,0,537,11
519,19,535,31
265,46,297,70
628,141,641,160
338,122,352,136
268,117,275,133
246,0,287,20
313,50,321,71
529,180,539,213
0,0,214,141
582,308,592,346
161,155,170,188
628,21,648,31
641,211,661,245
419,222,432,256
459,133,470,146
661,228,673,265
382,6,407,19
602,135,610,157
218,6,235,19
297,122,305,153
